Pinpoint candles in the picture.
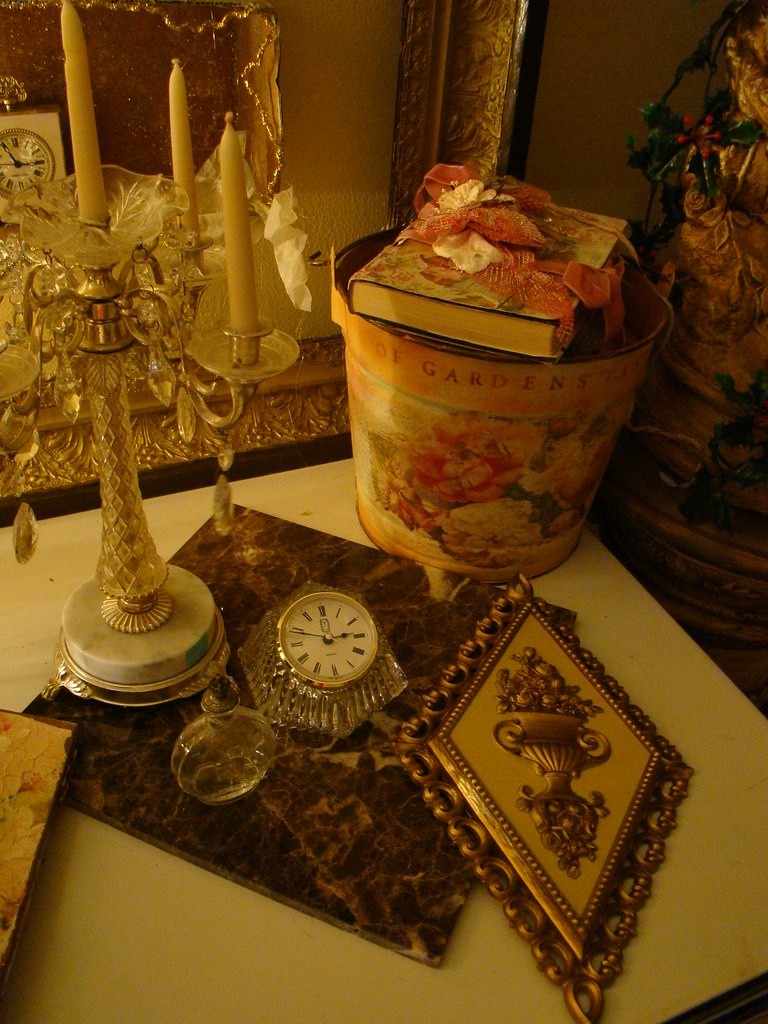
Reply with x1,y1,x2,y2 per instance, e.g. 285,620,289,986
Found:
170,59,199,244
60,0,106,225
220,111,258,329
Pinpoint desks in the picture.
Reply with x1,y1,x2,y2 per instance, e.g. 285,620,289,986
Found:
0,434,768,1024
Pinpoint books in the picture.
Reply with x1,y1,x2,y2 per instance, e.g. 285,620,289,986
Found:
347,208,632,368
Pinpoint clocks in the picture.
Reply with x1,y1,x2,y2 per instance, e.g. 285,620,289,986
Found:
1,105,70,223
238,580,409,739
